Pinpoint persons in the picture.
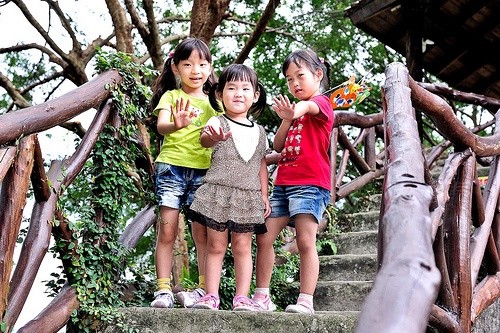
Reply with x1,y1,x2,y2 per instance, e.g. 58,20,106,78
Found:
150,38,225,309
251,49,335,314
194,64,271,311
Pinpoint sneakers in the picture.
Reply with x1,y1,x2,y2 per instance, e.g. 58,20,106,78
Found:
150,289,175,308
177,288,206,308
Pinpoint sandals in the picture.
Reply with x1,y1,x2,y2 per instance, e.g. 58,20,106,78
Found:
252,293,273,312
233,294,252,312
285,297,315,315
193,293,220,312
